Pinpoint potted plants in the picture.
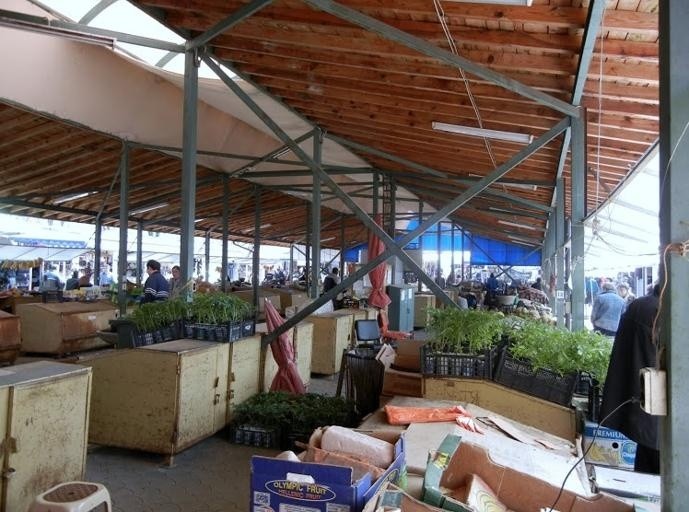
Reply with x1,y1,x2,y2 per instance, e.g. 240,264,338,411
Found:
420,306,612,406
124,293,254,347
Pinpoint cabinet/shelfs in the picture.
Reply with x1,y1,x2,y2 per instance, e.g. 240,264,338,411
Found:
0,358,92,511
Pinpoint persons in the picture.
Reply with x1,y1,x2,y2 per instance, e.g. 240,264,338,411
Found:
434,269,445,289
165,264,182,297
79,270,93,287
65,269,80,290
136,258,170,308
530,277,542,290
43,266,65,290
595,282,663,475
323,266,348,310
486,272,498,304
586,276,635,337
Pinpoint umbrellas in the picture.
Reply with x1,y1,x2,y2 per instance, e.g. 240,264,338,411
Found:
262,294,305,394
365,210,390,317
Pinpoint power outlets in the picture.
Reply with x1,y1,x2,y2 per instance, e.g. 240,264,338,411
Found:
637,366,667,415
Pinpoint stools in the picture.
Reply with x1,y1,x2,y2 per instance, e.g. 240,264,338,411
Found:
28,481,112,511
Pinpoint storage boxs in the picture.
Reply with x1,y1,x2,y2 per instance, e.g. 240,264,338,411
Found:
250,423,407,512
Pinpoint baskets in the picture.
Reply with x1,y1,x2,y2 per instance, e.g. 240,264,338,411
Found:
42,290,62,302
419,335,598,408
137,316,254,345
282,429,310,454
235,422,276,449
